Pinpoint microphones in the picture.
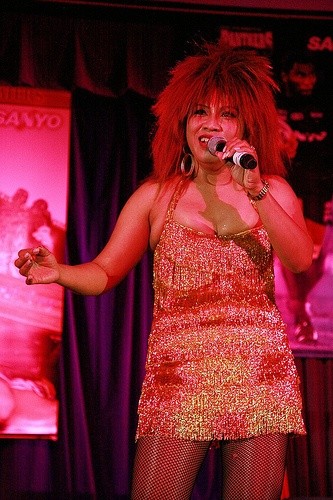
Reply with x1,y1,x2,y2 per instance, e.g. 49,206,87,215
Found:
208,136,257,170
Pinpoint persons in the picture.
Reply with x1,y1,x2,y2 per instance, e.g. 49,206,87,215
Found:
272,53,333,347
12,43,317,500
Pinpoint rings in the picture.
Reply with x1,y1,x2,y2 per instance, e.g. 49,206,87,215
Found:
249,145,256,150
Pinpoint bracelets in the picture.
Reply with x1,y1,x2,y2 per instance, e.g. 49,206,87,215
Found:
246,178,270,201
